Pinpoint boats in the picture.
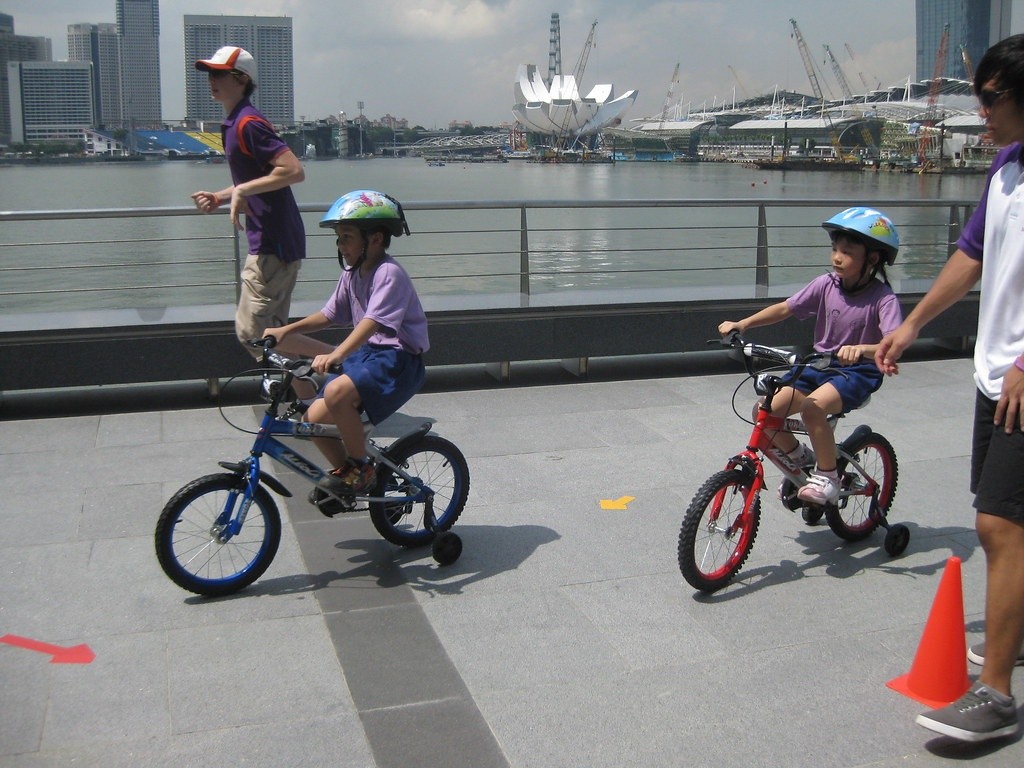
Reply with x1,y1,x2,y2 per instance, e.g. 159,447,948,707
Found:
752,155,870,173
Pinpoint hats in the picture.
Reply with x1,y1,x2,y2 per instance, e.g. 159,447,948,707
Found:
193,46,258,87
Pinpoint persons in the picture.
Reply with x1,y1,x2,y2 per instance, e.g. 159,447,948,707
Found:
261,189,432,510
876,30,1024,741
720,206,905,504
188,45,372,427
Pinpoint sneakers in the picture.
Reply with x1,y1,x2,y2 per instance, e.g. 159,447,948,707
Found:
798,468,841,507
967,637,1023,668
912,680,1018,744
273,399,312,424
327,455,377,494
776,444,816,496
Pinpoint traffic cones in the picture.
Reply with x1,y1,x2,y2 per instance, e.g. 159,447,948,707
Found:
885,558,977,715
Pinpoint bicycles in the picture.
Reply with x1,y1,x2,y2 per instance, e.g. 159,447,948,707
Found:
154,336,472,600
673,324,912,596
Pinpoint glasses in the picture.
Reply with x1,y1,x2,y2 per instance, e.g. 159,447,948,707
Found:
205,69,241,79
977,86,1012,107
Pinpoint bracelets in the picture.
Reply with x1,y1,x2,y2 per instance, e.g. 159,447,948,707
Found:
1014,357,1024,371
212,191,221,207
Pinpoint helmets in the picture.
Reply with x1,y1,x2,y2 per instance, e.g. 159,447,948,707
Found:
821,206,900,267
316,189,410,238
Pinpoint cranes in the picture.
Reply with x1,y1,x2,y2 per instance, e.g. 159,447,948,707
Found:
540,9,698,157
788,17,987,174
